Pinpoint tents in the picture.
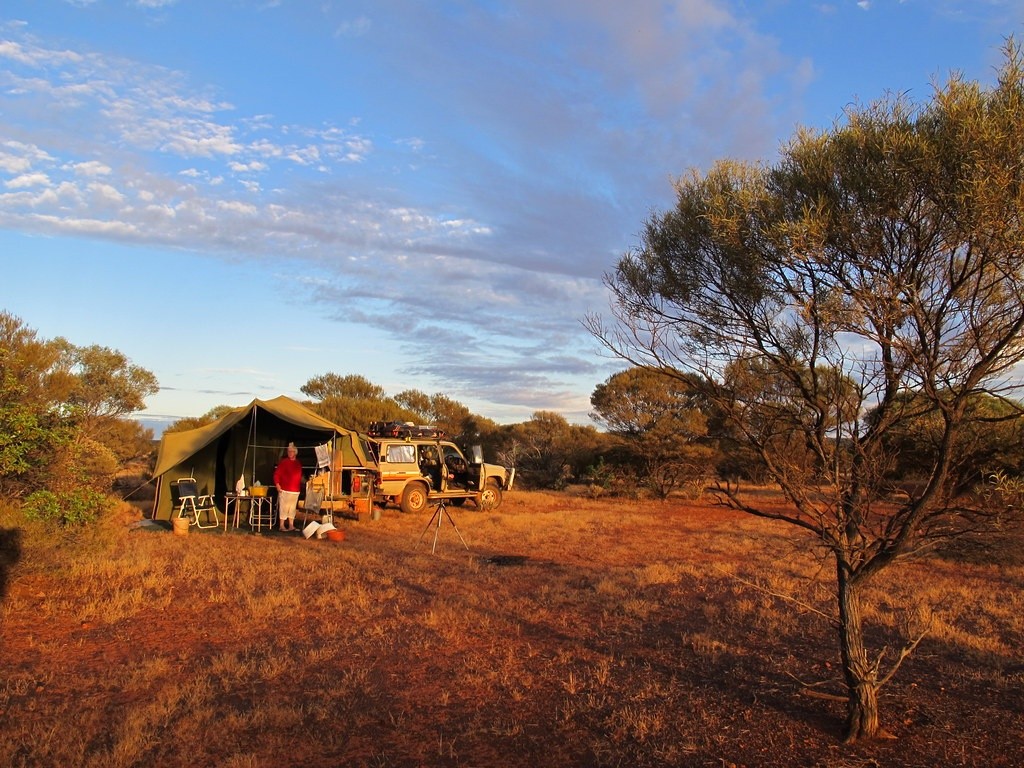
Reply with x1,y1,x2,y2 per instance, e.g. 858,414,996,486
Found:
124,395,368,522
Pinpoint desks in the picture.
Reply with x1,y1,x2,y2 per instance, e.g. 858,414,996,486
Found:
224,496,273,534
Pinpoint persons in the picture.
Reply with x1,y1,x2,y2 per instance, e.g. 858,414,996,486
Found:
273,443,302,531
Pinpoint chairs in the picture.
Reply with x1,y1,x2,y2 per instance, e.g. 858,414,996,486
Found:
249,486,280,527
418,451,456,481
177,478,218,529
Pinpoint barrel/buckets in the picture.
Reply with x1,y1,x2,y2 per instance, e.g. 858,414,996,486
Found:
172,518,191,535
372,510,381,520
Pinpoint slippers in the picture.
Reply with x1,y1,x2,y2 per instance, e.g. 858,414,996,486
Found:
279,528,290,532
289,527,300,532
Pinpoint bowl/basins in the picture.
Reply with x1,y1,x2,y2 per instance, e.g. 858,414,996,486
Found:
302,521,320,540
249,486,268,496
315,523,337,539
324,529,346,541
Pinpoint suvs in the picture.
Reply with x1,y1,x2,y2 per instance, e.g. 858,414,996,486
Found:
358,420,516,516
